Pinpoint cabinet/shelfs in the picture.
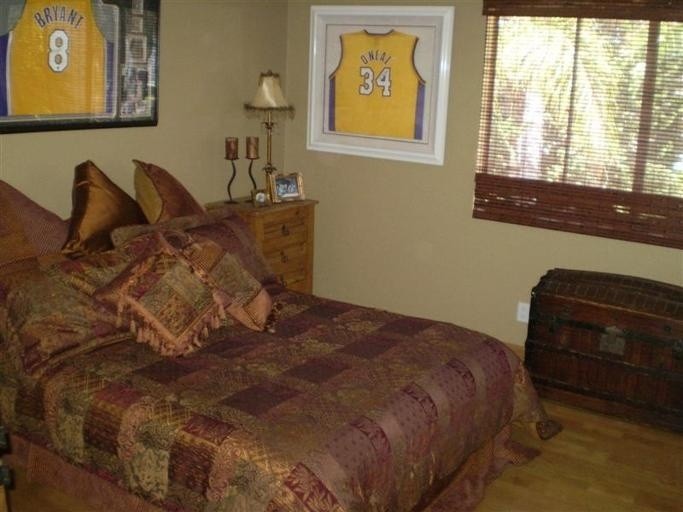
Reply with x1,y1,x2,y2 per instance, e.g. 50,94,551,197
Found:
523,264,682,432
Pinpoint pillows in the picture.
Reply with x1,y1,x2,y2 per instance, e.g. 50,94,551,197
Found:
1,157,286,392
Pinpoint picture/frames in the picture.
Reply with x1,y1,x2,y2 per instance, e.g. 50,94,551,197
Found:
270,170,305,202
307,4,454,166
0,1,160,127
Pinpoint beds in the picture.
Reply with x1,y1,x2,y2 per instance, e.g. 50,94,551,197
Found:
0,161,559,512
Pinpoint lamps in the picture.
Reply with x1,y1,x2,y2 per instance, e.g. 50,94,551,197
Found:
244,71,293,201
244,136,258,199
224,136,239,204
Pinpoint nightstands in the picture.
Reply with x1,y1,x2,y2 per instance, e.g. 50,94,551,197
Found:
205,195,319,297
